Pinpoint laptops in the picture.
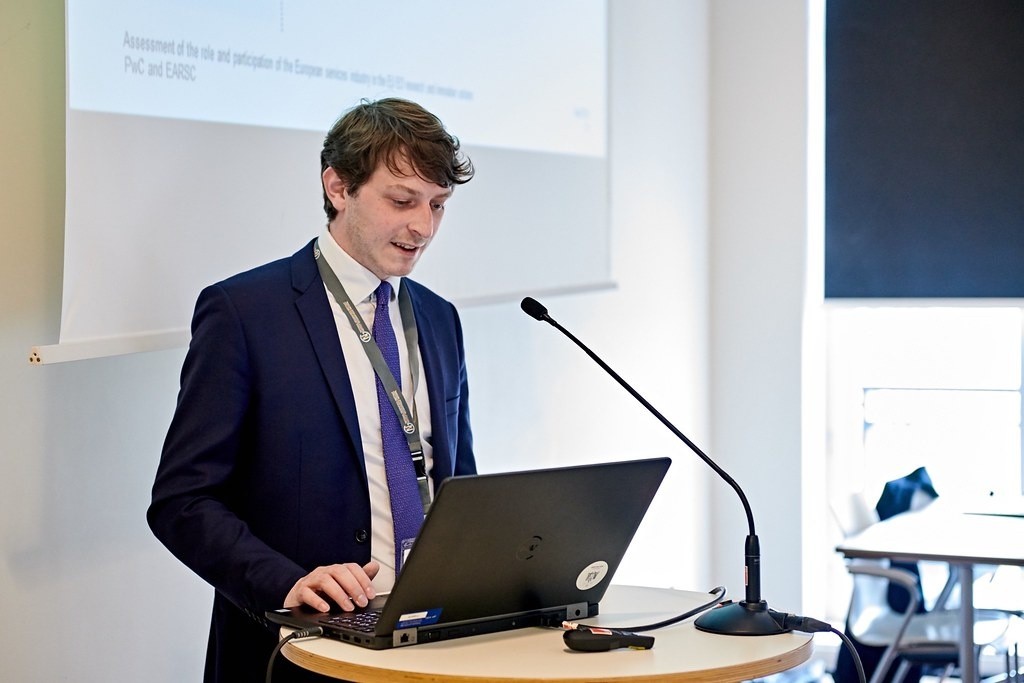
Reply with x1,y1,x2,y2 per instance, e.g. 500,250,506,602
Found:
266,457,671,650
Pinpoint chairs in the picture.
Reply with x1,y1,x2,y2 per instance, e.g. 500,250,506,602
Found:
875,466,1024,682
829,493,1009,683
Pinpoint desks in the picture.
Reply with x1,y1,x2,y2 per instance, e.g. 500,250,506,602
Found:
279,584,814,683
835,512,1024,683
923,490,1024,518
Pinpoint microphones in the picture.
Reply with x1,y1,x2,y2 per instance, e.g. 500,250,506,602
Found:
522,297,791,636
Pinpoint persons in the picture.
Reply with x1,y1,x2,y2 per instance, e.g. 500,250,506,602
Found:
145,99,479,683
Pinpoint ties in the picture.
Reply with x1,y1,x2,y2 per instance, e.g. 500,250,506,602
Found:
372,278,425,583
